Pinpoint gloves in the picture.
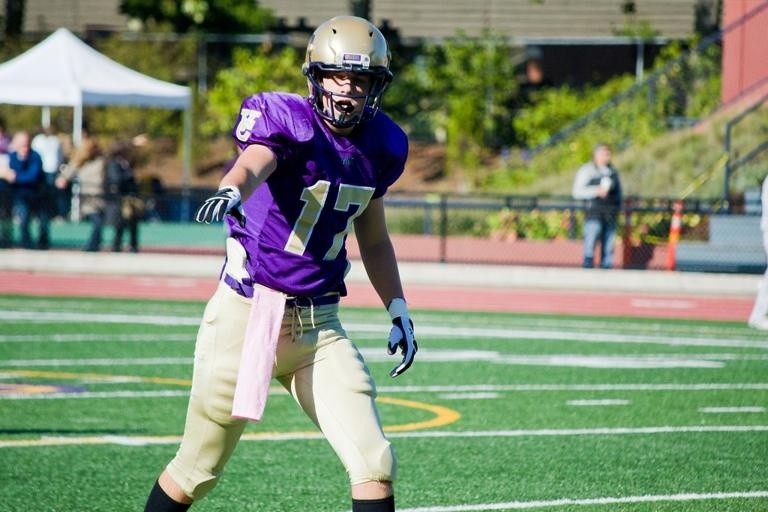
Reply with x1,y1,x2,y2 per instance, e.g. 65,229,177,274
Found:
382,296,421,378
193,186,249,230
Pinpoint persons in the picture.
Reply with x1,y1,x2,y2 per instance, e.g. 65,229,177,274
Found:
0,116,171,251
142,14,421,511
573,144,621,268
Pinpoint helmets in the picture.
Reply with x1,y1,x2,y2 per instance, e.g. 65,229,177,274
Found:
301,13,394,105
